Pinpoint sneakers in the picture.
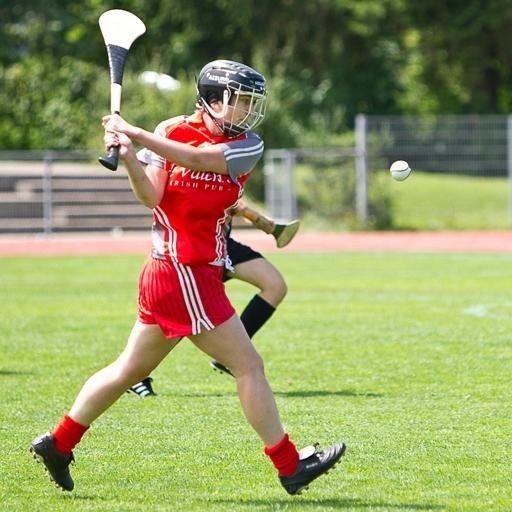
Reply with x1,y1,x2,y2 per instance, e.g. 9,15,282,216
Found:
209,358,236,376
279,442,346,496
125,377,157,398
29,430,75,491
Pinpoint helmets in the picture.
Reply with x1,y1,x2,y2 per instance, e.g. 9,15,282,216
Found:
196,59,269,139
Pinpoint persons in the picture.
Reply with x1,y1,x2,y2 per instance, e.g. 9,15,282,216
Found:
25,58,347,496
119,147,289,401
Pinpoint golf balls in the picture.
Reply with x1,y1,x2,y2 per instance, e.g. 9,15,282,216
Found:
390,160,410,180
